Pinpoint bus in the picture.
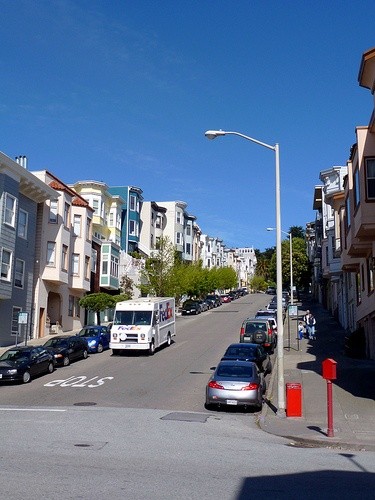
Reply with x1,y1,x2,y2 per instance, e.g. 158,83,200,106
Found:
110,297,175,356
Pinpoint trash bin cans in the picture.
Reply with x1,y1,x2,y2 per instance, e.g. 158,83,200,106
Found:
285,382,302,417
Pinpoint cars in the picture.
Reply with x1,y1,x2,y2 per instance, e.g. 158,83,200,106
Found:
197,287,249,312
181,301,201,316
42,335,89,367
76,326,111,354
220,343,273,375
0,345,55,385
207,359,267,413
264,291,289,324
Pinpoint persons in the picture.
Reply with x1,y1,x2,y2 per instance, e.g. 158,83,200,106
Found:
298,310,318,342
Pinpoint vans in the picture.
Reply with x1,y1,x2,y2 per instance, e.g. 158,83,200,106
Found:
266,286,276,296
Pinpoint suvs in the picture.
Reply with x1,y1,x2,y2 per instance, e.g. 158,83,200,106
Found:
255,316,278,336
256,309,277,323
240,320,277,352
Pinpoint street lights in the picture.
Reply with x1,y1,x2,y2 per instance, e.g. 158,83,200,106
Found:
266,227,294,307
204,128,286,418
